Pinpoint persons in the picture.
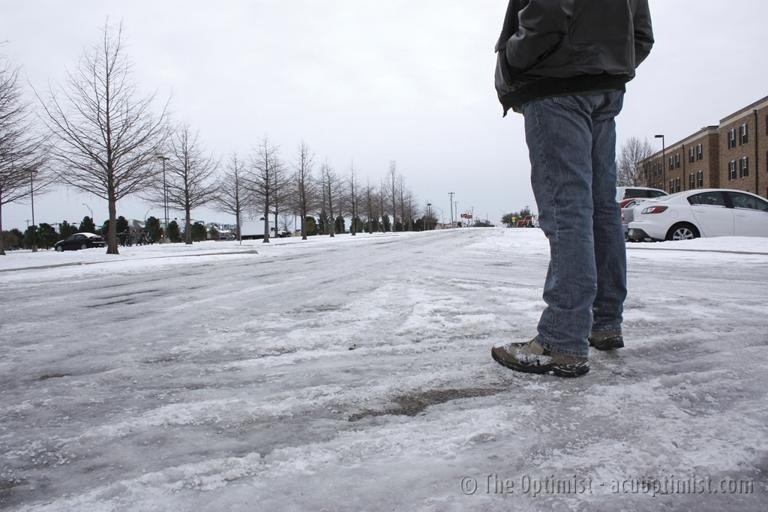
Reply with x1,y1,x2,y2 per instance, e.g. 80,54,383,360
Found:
491,0,655,378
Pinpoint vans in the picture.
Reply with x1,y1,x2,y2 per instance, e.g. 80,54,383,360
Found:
614,185,668,203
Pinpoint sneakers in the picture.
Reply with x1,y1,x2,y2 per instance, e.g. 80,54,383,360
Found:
587,330,624,350
492,338,591,377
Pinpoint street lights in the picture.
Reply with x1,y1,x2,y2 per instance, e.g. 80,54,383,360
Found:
448,192,454,228
156,155,172,244
426,203,431,214
22,167,40,254
81,202,94,218
653,134,666,191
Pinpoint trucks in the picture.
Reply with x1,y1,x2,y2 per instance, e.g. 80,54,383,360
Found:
239,217,287,239
173,217,234,241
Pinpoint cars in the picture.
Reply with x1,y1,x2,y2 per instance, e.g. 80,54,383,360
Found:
53,230,106,253
625,186,768,242
616,197,646,241
514,214,539,227
